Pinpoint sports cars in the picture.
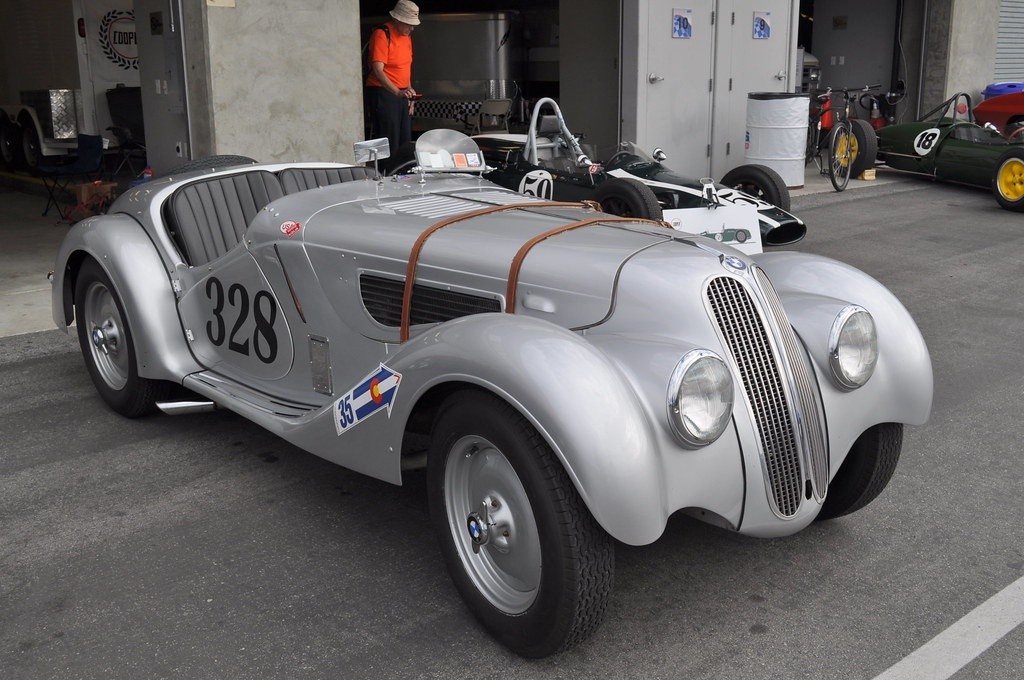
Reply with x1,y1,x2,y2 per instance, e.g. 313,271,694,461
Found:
394,98,807,249
874,92,1024,210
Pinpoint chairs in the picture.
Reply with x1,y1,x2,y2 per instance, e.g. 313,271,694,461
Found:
30,126,147,221
468,98,513,135
168,170,284,268
278,166,383,197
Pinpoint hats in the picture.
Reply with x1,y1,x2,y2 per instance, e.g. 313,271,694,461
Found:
390,0,420,25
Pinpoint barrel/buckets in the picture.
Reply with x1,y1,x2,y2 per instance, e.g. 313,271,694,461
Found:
744,92,810,190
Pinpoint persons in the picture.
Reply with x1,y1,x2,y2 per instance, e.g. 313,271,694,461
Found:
362,0,421,175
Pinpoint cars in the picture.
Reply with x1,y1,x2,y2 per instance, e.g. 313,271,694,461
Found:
47,128,935,663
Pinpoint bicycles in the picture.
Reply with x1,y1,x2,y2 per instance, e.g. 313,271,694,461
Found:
806,84,882,192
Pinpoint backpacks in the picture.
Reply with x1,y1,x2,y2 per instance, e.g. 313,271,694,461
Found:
362,25,390,74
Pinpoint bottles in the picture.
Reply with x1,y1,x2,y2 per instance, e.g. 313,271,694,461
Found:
143,164,152,179
